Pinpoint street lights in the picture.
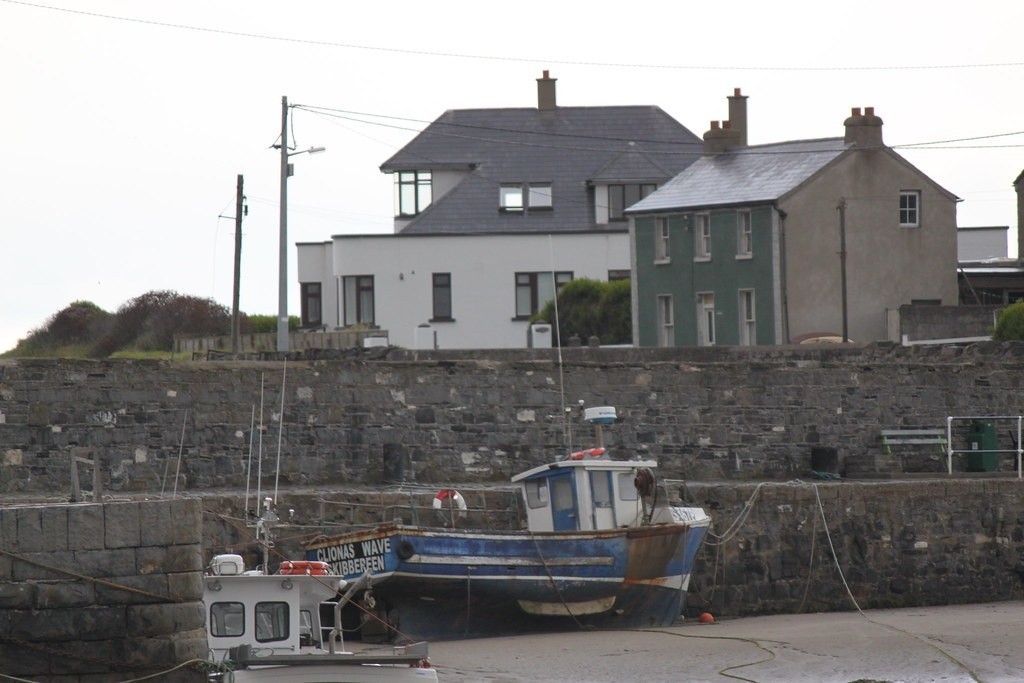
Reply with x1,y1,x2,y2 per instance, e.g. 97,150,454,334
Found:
276,146,327,352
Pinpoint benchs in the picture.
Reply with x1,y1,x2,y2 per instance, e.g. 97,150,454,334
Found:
879,428,960,473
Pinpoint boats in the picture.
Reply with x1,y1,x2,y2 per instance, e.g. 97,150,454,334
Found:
203,358,451,683
306,228,716,641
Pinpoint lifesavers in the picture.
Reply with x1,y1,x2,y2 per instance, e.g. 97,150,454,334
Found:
433,488,467,527
571,447,605,459
280,560,329,575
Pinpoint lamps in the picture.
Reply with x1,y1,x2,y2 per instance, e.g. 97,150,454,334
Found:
400,273,403,279
